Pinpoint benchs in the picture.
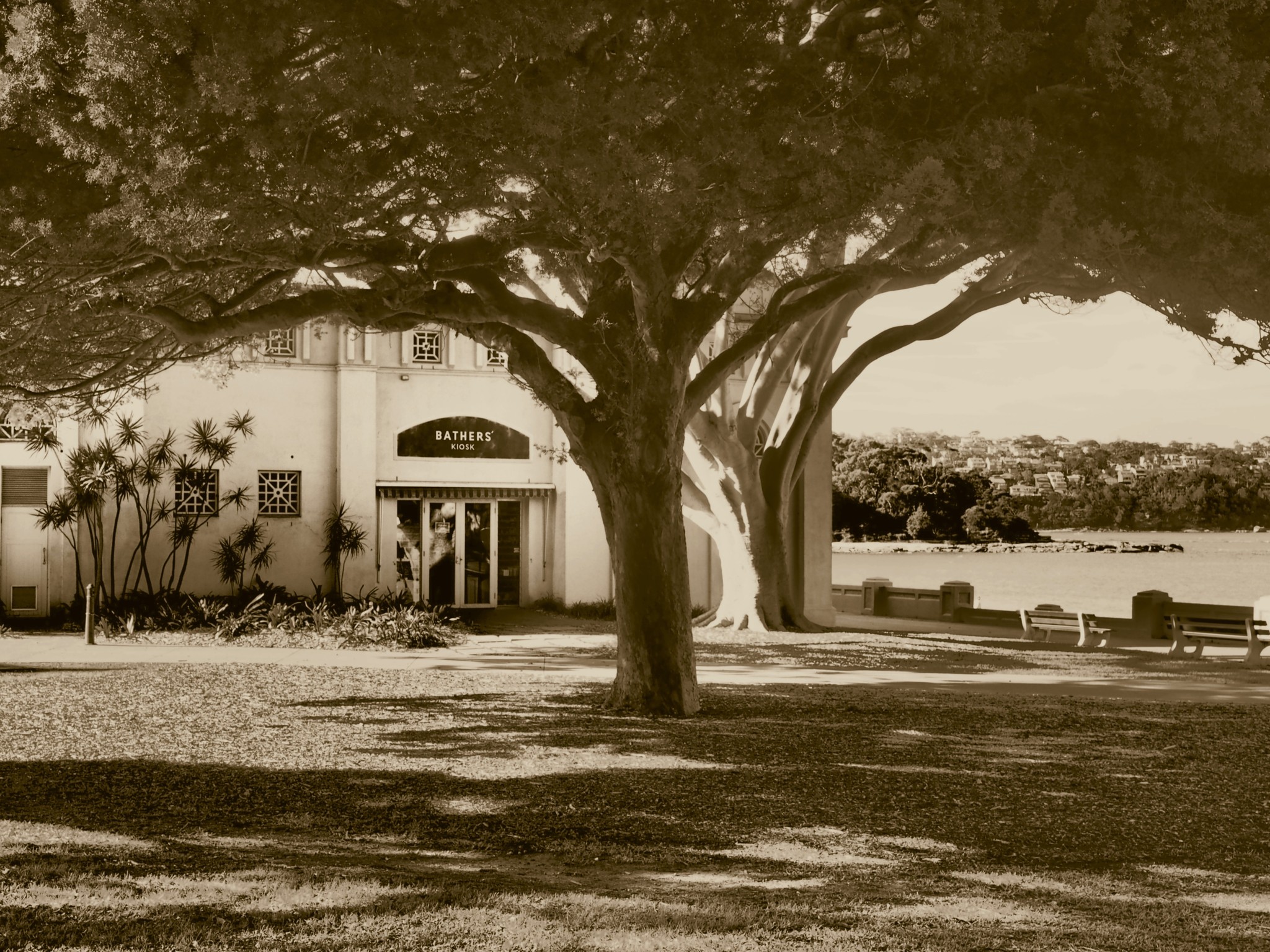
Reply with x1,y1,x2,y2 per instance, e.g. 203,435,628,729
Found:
1163,614,1270,665
1018,607,1113,649
